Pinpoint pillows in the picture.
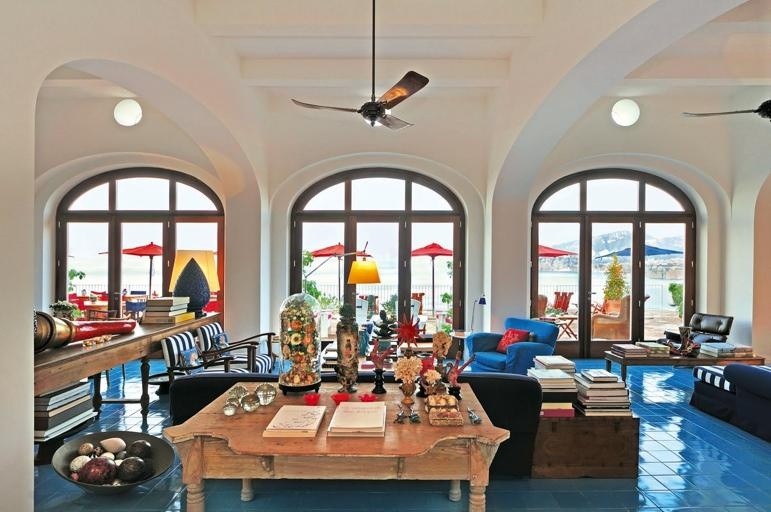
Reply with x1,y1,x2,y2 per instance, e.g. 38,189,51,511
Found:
497,328,529,354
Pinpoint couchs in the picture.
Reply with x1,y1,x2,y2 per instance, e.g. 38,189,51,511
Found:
170,372,542,481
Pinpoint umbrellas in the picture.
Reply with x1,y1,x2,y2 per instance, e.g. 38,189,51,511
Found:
411,242,454,319
537,244,576,260
100,241,174,299
307,243,372,308
594,244,685,258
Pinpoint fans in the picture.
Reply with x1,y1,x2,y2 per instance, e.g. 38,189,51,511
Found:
681,100,770,119
291,0,432,132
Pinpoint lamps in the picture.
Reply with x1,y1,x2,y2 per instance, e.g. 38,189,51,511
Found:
167,248,221,293
470,294,486,332
346,250,381,284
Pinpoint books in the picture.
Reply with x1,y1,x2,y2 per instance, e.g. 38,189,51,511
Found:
700,341,752,353
34,380,97,444
700,349,753,358
330,401,385,431
528,353,576,418
574,368,633,417
326,405,387,438
263,405,326,437
636,342,670,357
611,343,647,358
142,296,195,325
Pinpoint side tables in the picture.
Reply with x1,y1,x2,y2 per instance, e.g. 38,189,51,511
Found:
449,332,469,359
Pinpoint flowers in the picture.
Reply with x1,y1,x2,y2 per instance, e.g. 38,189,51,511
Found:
394,354,425,385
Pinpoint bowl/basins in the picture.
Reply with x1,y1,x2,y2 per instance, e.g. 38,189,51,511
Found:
51,430,175,496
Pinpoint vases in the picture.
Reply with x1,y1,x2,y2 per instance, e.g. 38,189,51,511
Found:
401,380,416,405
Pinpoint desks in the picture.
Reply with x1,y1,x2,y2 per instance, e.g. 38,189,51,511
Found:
556,315,578,340
34,312,221,465
162,381,510,512
604,351,766,389
532,412,641,480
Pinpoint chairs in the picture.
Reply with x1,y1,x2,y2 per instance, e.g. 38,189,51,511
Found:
410,292,425,314
68,290,127,317
356,298,368,326
663,313,734,368
360,295,378,315
160,322,277,416
395,298,427,328
86,308,126,387
688,364,770,444
591,295,631,340
553,291,572,312
466,317,559,376
